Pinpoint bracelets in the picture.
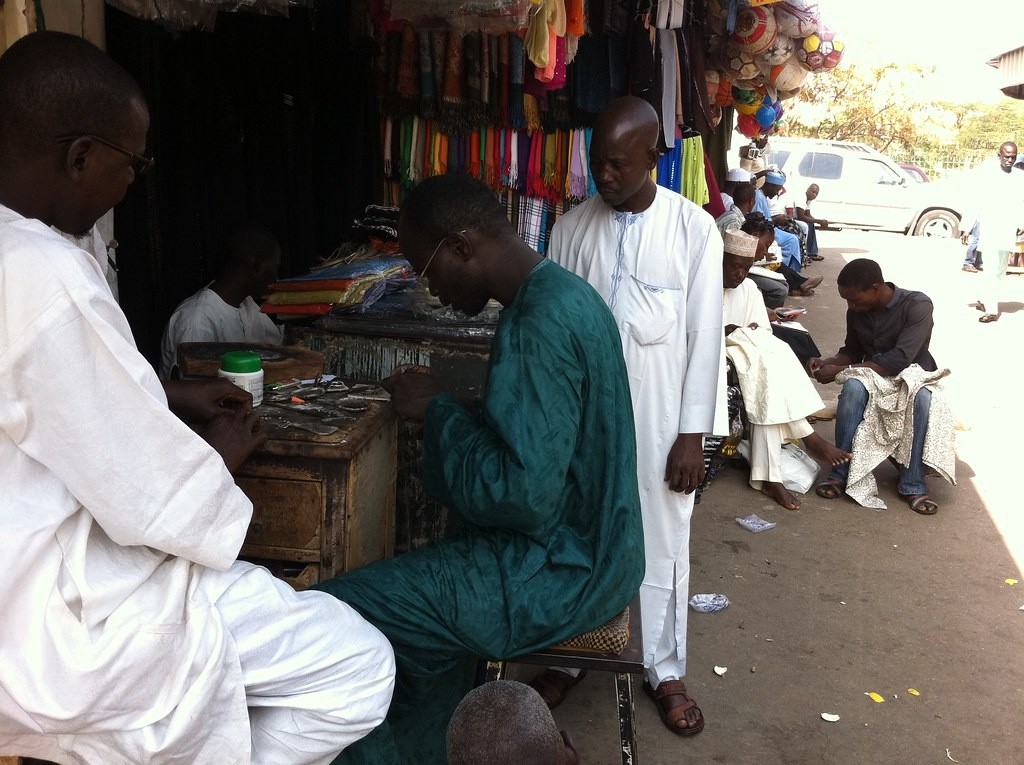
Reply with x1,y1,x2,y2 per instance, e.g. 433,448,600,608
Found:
849,365,851,368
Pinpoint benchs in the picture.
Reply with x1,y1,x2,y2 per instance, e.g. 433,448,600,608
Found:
474,589,644,765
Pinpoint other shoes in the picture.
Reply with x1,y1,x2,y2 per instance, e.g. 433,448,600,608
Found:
791,288,815,297
807,252,824,261
963,263,978,274
976,302,985,311
799,276,824,294
979,313,998,323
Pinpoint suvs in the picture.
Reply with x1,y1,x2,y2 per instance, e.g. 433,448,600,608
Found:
756,135,978,239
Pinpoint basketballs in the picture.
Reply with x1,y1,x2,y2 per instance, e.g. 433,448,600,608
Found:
712,74,736,109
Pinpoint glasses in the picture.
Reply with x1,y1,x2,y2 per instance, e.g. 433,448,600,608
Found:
416,226,468,288
56,131,156,176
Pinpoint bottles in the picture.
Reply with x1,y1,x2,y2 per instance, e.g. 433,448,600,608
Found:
218,351,264,408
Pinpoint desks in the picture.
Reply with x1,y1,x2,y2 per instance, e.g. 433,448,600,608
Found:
232,369,398,593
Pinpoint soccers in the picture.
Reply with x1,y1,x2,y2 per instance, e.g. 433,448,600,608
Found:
705,0,847,104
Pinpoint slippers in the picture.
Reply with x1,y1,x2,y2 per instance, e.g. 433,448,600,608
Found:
527,665,587,709
815,473,843,500
643,677,703,736
898,489,940,516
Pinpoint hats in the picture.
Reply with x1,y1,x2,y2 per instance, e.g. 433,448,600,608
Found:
766,171,786,185
729,167,751,182
721,227,760,259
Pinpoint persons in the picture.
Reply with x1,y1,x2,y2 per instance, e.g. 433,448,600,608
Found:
722,229,853,510
445,679,581,765
961,161,1024,274
312,173,646,765
958,141,1024,323
158,220,285,380
0,30,397,765
715,134,828,367
525,95,730,737
805,258,938,515
